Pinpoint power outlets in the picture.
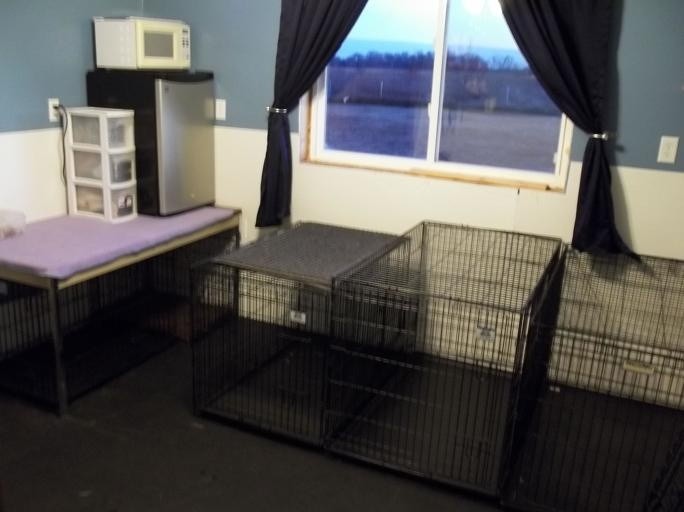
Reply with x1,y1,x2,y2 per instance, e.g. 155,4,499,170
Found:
48,98,60,122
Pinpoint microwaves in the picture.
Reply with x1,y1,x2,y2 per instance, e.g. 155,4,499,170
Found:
93,16,191,71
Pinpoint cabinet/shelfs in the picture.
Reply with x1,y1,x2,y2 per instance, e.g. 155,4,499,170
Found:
63,106,138,224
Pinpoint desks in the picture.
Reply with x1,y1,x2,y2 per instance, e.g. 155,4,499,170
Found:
0,208,245,414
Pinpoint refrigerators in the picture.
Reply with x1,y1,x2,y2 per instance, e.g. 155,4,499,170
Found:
82,70,214,218
